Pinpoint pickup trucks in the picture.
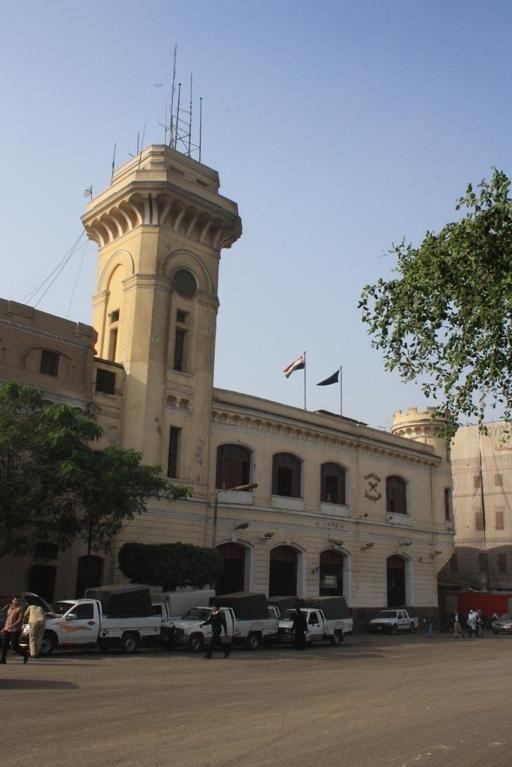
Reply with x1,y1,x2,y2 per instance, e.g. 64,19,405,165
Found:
367,609,420,634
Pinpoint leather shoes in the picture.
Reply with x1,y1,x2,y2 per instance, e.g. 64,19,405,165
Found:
0,652,29,664
203,650,230,658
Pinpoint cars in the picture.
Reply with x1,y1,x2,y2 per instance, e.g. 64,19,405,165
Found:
491,611,512,634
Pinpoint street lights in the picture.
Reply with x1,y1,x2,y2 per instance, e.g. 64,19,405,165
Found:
212,483,257,585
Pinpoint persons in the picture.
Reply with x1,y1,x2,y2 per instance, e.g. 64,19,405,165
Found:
1,595,46,663
290,606,308,651
423,606,500,639
199,603,230,659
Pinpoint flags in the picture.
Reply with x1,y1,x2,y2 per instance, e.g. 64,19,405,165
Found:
316,370,340,386
282,355,305,378
